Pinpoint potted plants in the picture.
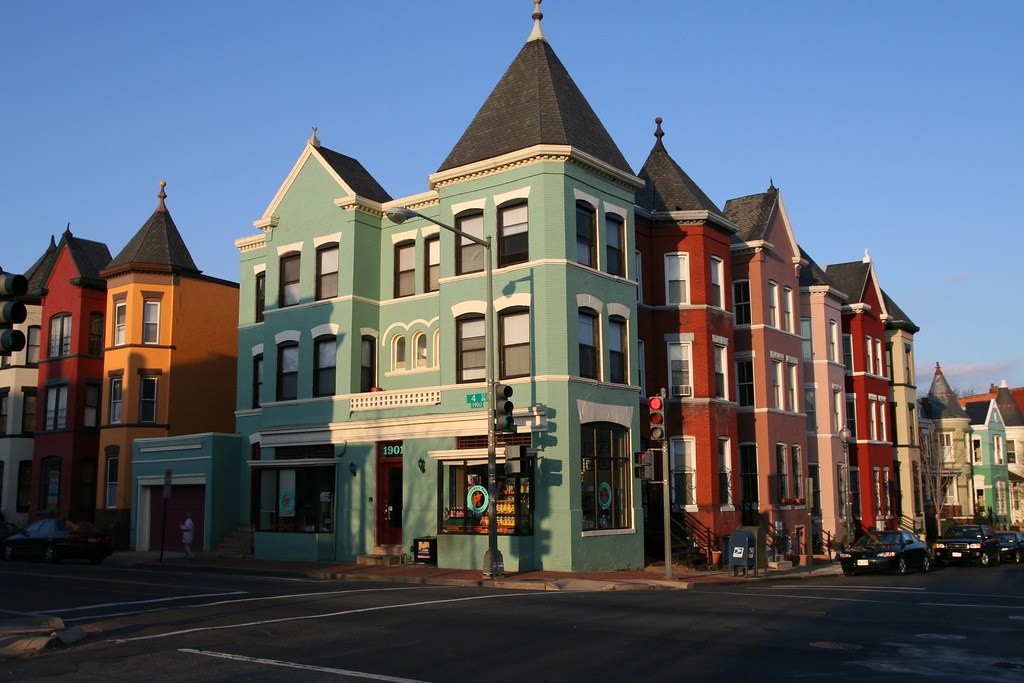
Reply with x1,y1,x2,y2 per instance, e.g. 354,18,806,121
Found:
707,535,722,564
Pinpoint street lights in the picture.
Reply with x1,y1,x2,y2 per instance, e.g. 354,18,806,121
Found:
384,207,504,579
962,425,976,516
839,425,854,548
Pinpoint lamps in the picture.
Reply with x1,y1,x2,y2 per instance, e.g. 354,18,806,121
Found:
349,461,356,477
418,457,425,473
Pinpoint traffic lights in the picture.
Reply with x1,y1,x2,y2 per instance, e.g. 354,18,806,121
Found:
0,266,28,356
648,396,666,441
494,381,515,432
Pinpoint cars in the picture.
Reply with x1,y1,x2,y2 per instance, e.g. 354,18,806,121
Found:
0,517,115,564
0,520,20,542
995,531,1024,564
839,529,932,578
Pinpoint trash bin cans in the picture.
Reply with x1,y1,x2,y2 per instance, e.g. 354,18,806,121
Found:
414,536,438,567
729,531,756,577
735,525,768,576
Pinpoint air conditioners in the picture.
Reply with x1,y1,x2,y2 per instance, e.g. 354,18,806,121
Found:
672,385,691,396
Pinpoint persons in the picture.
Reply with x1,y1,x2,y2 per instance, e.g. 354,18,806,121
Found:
180,512,195,561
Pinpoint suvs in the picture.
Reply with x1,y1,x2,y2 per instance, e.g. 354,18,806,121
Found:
934,524,1000,569
